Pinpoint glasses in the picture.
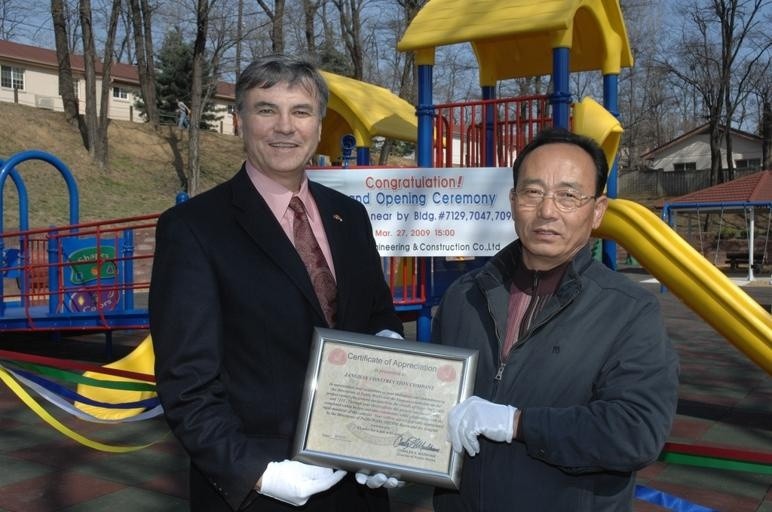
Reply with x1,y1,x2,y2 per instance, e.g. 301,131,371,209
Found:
514,187,597,212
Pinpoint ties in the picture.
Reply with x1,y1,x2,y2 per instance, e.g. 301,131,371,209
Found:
289,196,338,329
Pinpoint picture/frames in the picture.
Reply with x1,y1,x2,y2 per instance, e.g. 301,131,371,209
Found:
289,328,479,491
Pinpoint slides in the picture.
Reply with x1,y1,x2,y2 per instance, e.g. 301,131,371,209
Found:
75,332,173,423
593,194,772,370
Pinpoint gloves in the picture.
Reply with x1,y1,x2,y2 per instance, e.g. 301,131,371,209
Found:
356,473,405,489
448,395,518,456
256,459,346,506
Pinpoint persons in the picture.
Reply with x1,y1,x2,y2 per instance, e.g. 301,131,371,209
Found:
354,125,681,512
175,97,191,129
147,51,411,512
